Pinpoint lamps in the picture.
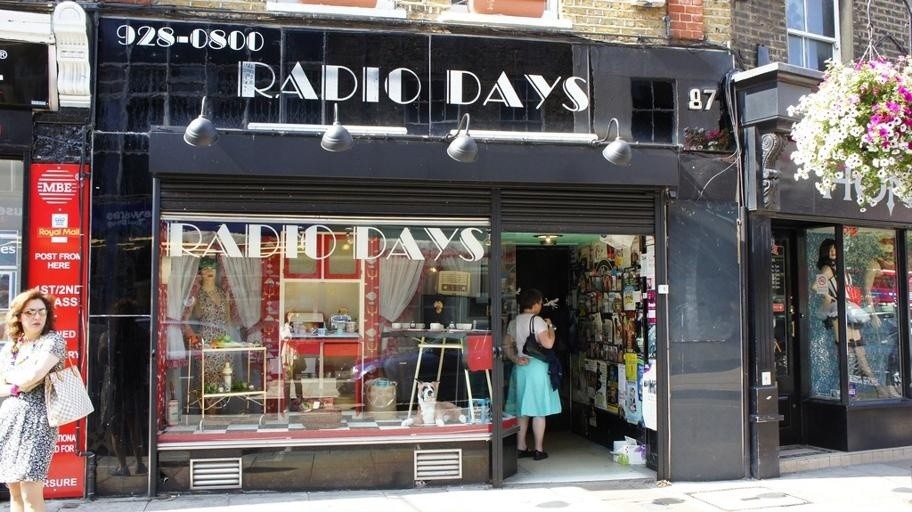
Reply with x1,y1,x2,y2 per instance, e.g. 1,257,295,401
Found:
320,102,355,153
446,113,479,163
590,117,632,167
183,96,220,147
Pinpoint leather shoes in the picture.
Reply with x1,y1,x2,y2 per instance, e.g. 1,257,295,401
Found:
515,448,533,457
532,450,548,461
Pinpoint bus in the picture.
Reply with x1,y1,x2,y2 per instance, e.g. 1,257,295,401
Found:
849,268,912,315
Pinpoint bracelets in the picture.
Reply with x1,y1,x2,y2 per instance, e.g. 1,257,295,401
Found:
549,324,553,327
10,384,19,396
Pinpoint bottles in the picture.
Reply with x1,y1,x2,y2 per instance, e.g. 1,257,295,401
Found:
168,399,179,426
223,361,233,392
448,320,455,334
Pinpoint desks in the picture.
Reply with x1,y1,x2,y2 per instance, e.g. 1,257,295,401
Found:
277,231,366,412
381,329,493,424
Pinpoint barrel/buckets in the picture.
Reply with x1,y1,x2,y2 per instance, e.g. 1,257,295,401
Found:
364,378,398,419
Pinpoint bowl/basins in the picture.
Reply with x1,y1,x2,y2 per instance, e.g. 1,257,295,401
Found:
456,324,472,330
330,315,351,329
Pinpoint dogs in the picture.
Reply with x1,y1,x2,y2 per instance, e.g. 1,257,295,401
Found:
400,379,467,427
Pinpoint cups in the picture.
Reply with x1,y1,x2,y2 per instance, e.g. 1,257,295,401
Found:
391,322,445,330
290,321,303,335
338,320,359,334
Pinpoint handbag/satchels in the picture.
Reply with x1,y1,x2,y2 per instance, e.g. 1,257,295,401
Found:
44,364,96,428
523,315,556,364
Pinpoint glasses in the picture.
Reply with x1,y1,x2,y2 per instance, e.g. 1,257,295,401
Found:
24,309,47,317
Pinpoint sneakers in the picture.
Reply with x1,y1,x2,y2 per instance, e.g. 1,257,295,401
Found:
110,468,131,476
134,463,147,474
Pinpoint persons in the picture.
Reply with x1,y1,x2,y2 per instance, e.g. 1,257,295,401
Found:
96,299,148,476
182,256,236,416
812,239,890,399
863,233,902,397
502,289,563,461
0,289,69,511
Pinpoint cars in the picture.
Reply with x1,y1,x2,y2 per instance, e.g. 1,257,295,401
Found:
339,330,492,411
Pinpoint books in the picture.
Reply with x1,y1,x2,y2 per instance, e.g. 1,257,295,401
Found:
571,269,640,364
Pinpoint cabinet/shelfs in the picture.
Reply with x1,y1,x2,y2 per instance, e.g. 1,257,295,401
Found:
187,339,267,417
